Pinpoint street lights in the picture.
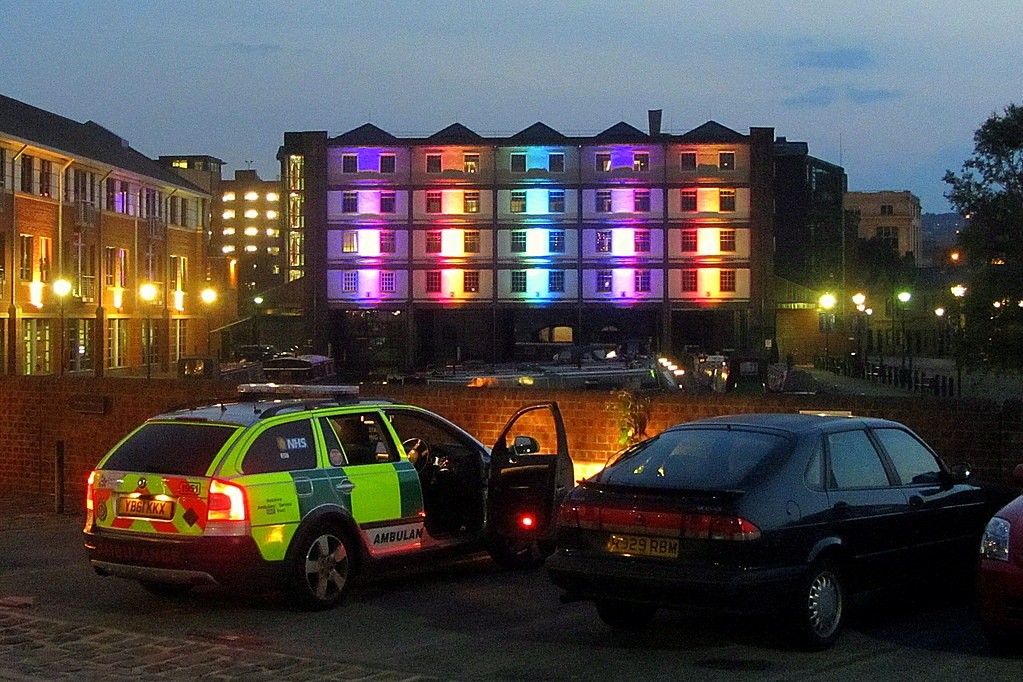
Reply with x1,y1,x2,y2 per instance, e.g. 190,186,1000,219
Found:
897,291,911,388
818,292,834,372
140,283,157,379
951,283,967,399
201,288,215,359
852,292,873,378
935,308,944,357
53,277,72,374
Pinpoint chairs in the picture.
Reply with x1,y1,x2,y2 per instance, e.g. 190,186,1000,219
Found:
340,419,378,466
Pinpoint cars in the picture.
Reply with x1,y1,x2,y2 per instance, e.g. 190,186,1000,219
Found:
233,345,283,363
680,344,767,394
545,413,989,653
979,494,1023,608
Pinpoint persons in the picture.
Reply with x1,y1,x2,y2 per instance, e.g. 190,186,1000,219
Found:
339,420,467,532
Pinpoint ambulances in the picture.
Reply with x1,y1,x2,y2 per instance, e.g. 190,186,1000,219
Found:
83,384,574,608
